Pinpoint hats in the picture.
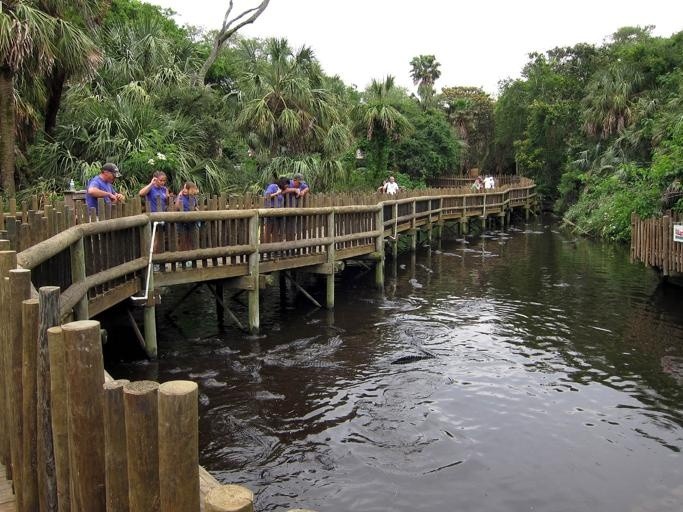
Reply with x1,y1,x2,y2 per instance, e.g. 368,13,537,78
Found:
103,162,123,178
294,173,306,183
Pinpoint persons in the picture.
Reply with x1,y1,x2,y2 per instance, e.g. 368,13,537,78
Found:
174,181,200,268
85,162,126,222
382,176,399,195
139,170,169,271
286,173,310,207
475,174,494,189
262,177,290,258
378,179,387,193
